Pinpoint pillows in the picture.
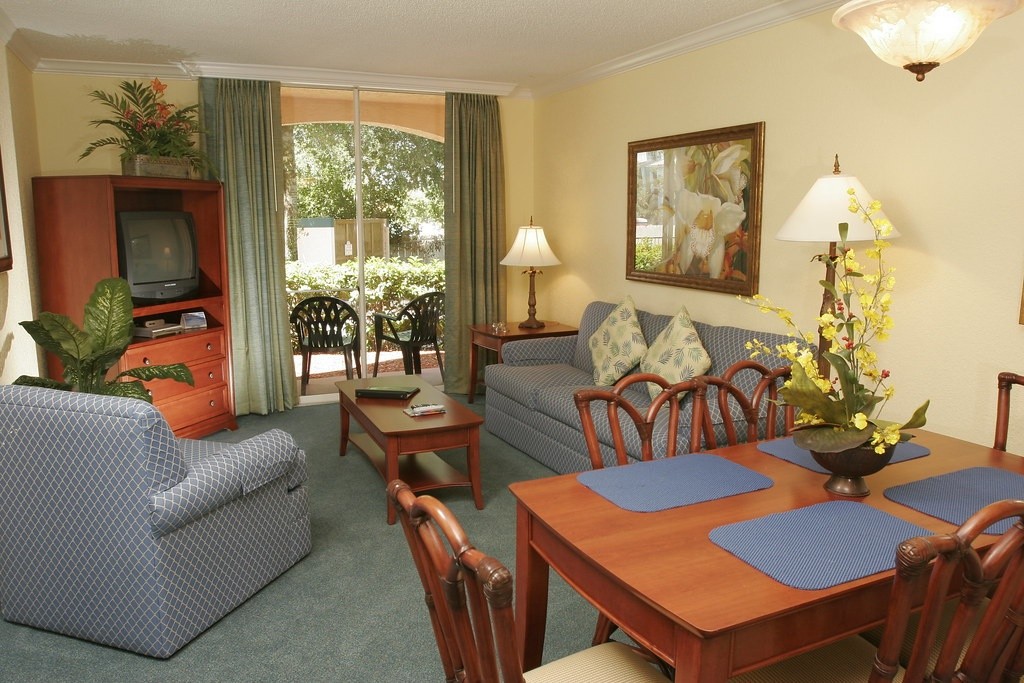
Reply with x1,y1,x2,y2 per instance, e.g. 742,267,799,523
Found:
640,305,712,408
588,297,648,387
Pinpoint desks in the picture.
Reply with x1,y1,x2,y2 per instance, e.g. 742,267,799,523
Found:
508,422,1024,683
335,375,484,524
467,320,579,403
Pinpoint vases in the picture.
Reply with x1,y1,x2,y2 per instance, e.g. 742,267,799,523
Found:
810,442,895,497
122,154,191,176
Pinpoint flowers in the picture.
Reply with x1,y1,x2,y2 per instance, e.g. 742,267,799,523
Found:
737,187,931,454
87,77,220,178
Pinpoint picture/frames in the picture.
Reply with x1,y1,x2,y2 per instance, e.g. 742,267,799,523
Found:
625,121,765,298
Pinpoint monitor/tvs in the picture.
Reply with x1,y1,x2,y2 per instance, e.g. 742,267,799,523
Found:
116,211,200,304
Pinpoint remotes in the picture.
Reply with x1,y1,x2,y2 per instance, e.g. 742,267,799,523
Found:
412,403,445,414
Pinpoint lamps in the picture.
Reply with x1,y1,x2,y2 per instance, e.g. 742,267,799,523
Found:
776,154,901,379
501,216,561,330
833,0,1024,82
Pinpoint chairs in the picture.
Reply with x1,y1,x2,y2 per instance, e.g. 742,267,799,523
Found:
290,296,360,395
387,361,1024,683
372,292,446,382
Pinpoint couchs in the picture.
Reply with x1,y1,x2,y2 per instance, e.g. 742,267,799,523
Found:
0,385,314,656
484,302,819,476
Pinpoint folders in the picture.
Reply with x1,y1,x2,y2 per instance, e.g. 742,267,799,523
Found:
355,386,419,399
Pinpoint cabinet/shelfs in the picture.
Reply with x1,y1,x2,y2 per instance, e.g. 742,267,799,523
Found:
32,175,239,439
